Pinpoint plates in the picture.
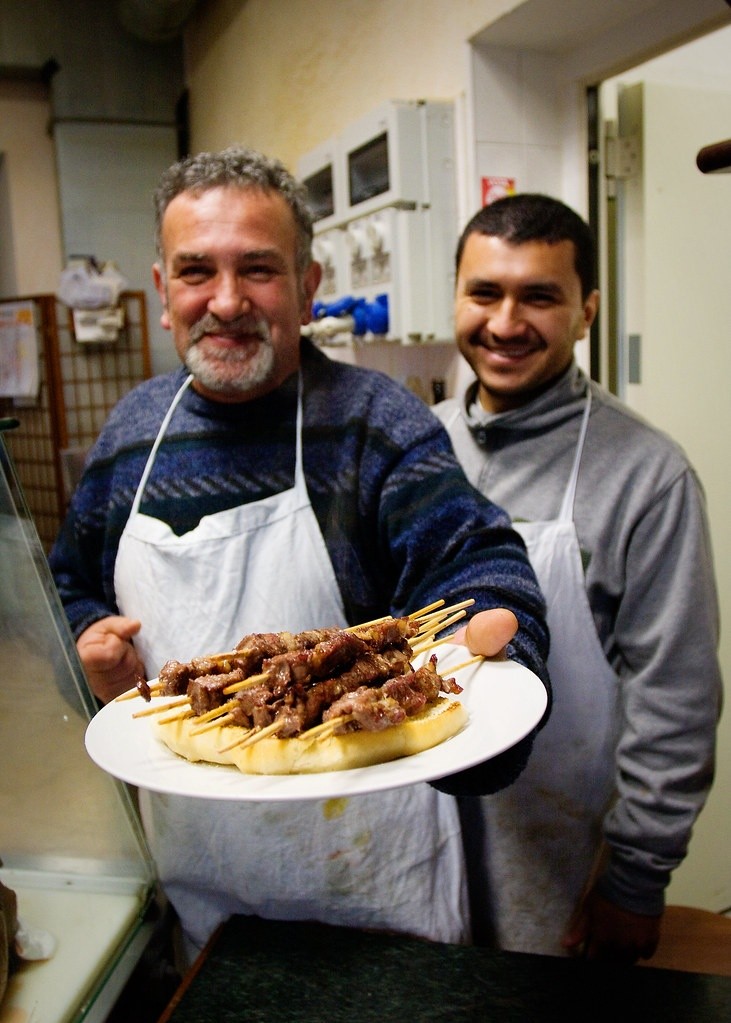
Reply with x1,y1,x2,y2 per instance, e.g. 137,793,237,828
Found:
84,643,548,800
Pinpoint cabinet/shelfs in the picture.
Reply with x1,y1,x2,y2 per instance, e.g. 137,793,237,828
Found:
297,98,459,348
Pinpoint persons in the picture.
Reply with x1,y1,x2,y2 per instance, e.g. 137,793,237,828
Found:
426,193,724,968
45,146,555,978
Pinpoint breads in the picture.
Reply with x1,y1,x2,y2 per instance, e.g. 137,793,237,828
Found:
154,687,467,775
